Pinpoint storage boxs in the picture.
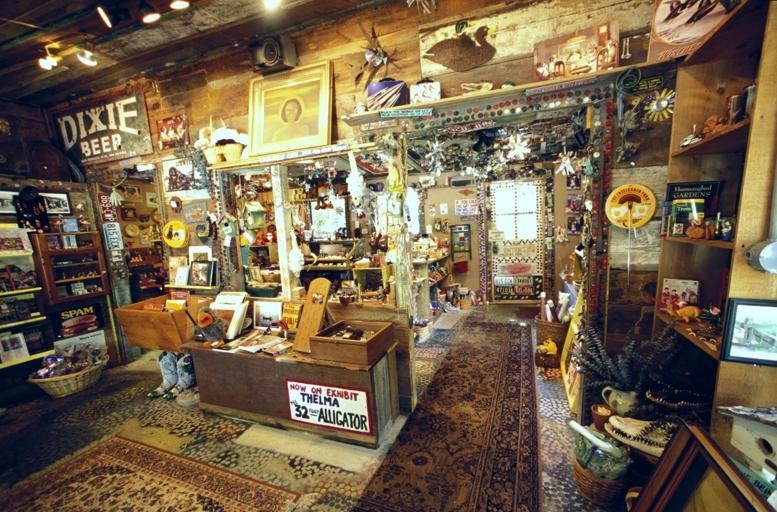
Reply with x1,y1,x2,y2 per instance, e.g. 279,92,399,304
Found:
309,319,394,367
113,291,217,351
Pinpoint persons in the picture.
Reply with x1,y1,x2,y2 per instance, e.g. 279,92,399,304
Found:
533,36,617,83
157,114,191,153
270,97,312,146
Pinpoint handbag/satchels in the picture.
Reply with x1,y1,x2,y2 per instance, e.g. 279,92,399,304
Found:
409,77,441,104
367,77,408,111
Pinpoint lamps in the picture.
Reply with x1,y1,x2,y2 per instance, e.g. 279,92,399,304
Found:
38,1,190,70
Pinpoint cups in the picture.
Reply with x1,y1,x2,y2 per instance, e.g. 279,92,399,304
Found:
591,403,615,431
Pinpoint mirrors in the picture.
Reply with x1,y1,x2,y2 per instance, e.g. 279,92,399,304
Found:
486,176,549,303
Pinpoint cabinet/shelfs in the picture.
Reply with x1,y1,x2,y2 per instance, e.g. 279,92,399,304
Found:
0,223,111,369
412,252,453,323
643,3,776,435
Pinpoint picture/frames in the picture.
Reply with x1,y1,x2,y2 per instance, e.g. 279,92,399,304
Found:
0,189,72,216
247,59,335,157
252,300,283,332
719,297,776,368
119,183,158,220
186,245,214,286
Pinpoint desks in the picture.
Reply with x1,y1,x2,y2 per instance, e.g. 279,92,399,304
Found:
352,265,390,292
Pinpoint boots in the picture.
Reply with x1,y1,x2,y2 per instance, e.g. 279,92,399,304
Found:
163,353,197,399
146,351,178,399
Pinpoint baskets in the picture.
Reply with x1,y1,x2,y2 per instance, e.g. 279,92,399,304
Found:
574,456,630,508
220,143,245,162
534,313,570,368
26,353,111,400
202,147,224,165
